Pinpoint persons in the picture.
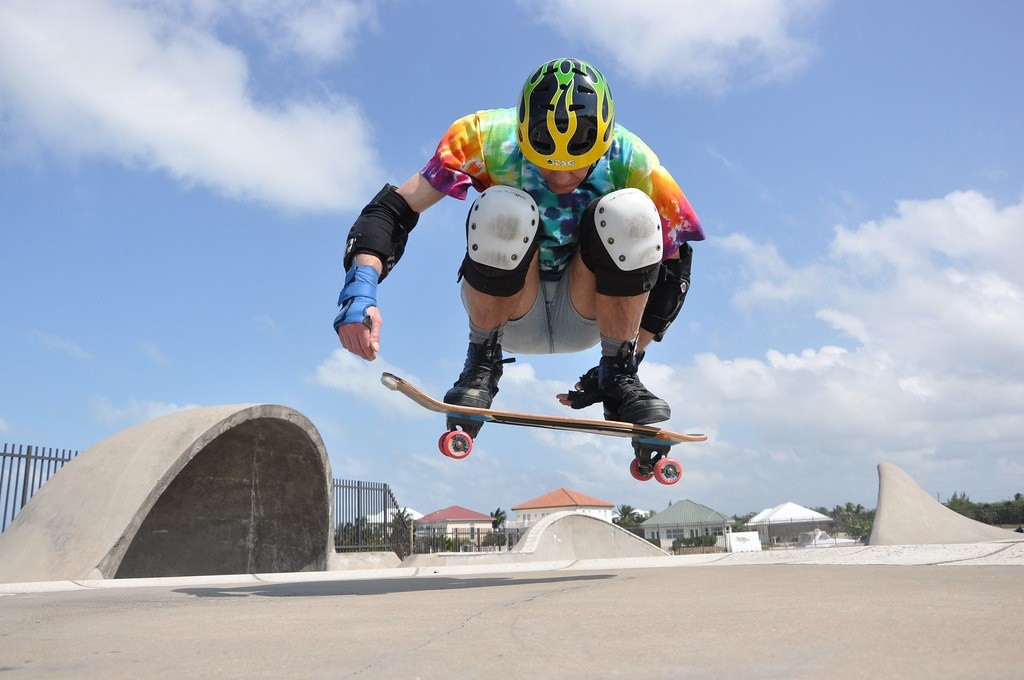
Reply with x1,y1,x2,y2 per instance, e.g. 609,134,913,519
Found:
333,58,706,424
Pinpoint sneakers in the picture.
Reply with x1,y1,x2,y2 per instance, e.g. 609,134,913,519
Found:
599,340,670,426
443,324,516,409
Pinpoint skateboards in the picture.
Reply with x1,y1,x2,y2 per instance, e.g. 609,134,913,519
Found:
381,372,708,485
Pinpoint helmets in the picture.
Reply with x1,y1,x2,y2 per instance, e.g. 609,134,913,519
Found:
516,58,615,170
